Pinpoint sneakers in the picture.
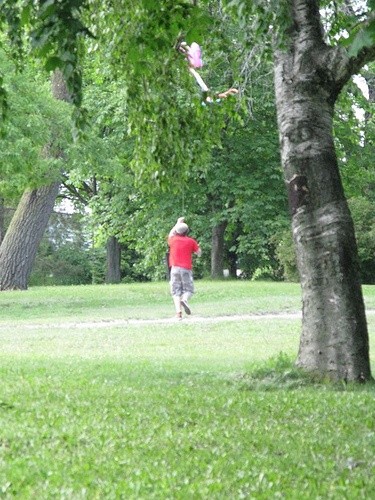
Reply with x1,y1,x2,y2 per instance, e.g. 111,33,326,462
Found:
180,300,191,315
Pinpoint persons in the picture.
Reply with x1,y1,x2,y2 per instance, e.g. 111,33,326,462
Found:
167,216,203,323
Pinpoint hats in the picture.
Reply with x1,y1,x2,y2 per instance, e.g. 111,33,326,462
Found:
175,223,188,235
